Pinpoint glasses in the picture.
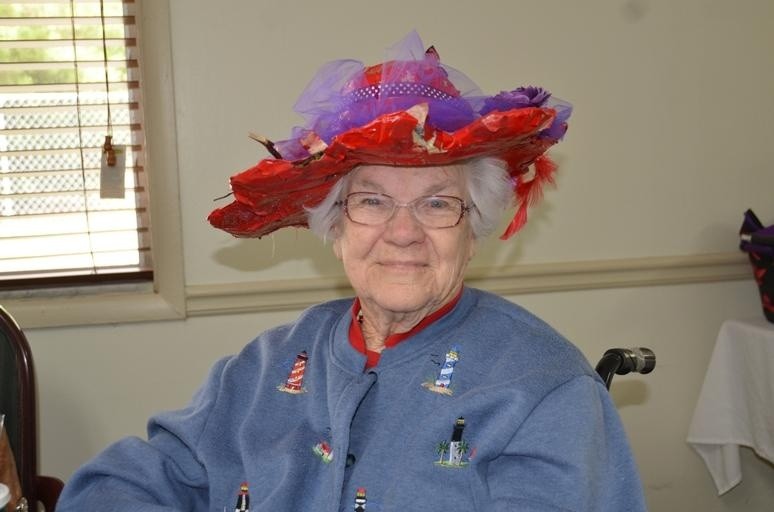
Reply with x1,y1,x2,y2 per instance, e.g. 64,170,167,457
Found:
336,191,475,229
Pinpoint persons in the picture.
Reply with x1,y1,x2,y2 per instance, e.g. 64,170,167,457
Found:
55,26,647,511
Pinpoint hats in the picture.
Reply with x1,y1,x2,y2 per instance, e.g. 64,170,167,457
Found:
207,58,568,237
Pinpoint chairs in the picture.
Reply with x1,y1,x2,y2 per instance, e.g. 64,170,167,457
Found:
0,304,65,512
